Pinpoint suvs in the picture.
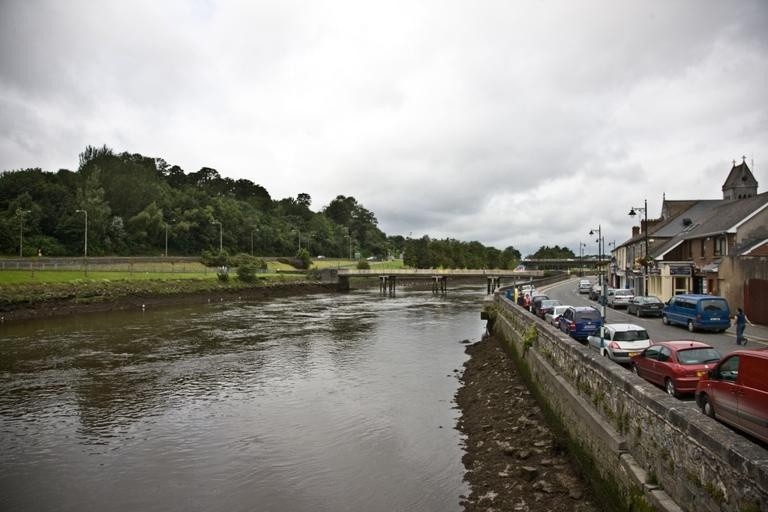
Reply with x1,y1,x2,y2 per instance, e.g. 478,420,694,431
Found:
607,288,635,310
585,322,653,365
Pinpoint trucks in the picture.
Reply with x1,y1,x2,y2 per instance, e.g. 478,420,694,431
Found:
661,292,733,334
695,346,768,448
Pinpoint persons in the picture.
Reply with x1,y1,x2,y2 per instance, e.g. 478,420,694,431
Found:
523,293,532,310
732,306,755,346
514,285,523,306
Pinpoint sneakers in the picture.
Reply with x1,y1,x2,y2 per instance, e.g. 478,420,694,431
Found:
737,341,741,345
743,338,747,345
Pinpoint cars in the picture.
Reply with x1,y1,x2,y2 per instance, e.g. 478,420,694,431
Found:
505,278,612,343
624,295,664,319
381,256,395,261
315,254,325,260
629,338,722,399
366,256,379,261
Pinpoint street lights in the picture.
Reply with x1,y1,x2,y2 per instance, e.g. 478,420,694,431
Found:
211,221,223,252
291,229,302,251
165,218,176,257
578,224,615,303
343,236,352,260
75,209,89,259
251,228,258,257
19,210,32,258
628,198,648,298
